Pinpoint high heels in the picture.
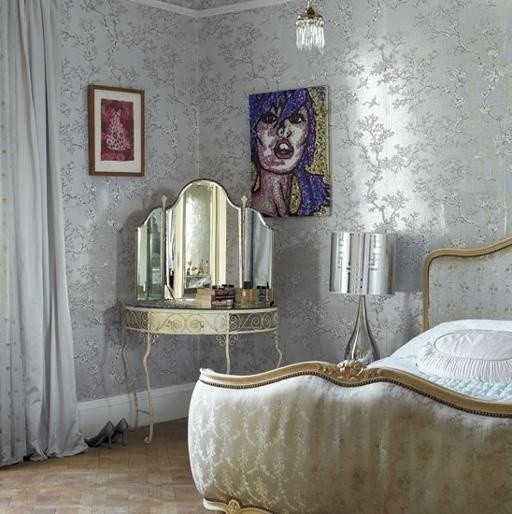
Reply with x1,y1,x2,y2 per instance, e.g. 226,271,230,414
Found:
85,418,128,449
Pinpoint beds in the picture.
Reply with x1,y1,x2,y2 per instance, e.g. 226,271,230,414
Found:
187,233,511,512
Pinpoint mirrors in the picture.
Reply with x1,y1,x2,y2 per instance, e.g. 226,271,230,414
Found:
133,178,274,302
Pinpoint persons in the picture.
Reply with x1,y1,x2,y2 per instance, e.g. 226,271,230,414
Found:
249,88,331,218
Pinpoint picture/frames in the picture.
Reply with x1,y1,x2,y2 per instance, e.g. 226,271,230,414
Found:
85,84,145,177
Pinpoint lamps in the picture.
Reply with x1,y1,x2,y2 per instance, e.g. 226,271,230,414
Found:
295,0,325,54
326,228,398,367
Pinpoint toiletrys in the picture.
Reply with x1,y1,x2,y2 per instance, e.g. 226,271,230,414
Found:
196,279,271,308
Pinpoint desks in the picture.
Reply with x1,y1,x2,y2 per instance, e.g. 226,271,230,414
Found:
120,299,281,444
